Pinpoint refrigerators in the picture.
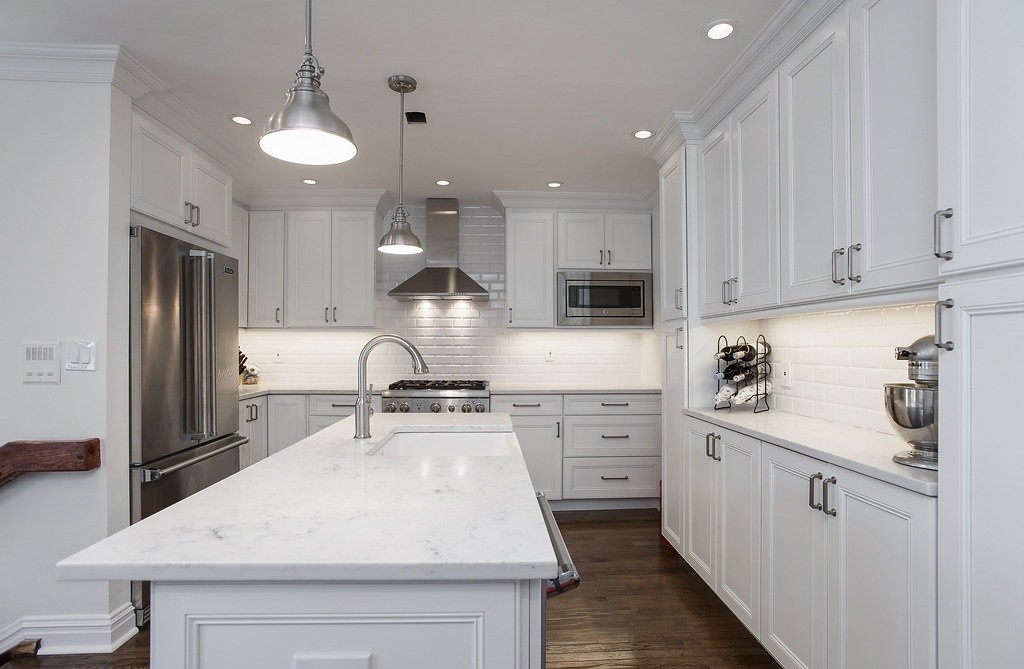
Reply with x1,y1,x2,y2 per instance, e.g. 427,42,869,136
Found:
128,223,251,627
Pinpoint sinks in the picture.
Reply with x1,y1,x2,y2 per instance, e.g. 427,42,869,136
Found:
370,431,523,457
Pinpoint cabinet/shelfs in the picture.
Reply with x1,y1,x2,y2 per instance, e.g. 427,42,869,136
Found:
232,204,384,329
488,394,661,513
236,394,382,471
503,208,651,329
131,101,233,249
643,0,1024,669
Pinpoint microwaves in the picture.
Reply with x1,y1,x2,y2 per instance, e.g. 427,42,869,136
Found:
557,269,652,327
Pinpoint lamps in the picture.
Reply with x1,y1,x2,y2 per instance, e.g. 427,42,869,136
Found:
257,0,357,165
377,75,424,255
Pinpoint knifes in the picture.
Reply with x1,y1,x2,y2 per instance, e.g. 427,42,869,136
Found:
239,346,248,375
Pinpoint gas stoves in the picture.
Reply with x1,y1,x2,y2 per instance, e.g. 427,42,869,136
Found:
382,379,491,414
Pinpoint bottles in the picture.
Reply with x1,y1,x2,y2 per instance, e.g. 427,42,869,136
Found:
713,346,739,361
732,342,771,362
733,361,771,382
730,379,774,405
712,361,752,380
714,381,746,404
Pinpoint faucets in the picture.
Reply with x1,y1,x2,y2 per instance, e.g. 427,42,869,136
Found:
353,334,430,440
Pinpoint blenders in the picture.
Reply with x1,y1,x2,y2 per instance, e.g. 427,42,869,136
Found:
883,335,938,470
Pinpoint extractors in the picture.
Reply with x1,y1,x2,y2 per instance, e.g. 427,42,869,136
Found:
386,198,490,303
